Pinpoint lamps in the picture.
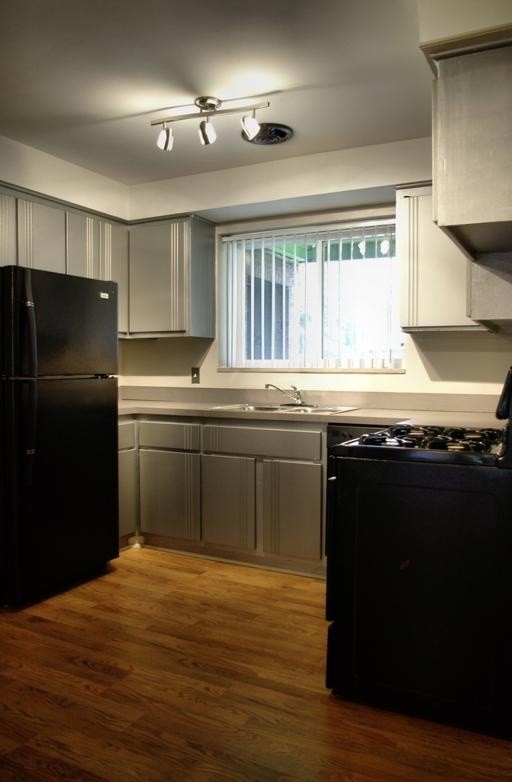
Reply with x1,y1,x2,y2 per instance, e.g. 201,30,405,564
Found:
150,95,271,153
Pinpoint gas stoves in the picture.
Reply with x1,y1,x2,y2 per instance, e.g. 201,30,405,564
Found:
328,423,511,463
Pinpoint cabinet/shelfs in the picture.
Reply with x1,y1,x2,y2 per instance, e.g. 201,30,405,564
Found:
66,212,129,335
137,422,202,542
397,195,480,328
203,425,322,562
1,195,66,275
119,422,138,539
129,222,188,334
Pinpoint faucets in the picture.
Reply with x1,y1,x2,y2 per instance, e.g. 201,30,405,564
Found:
265,384,303,405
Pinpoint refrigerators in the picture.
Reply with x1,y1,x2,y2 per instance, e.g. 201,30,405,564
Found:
0,265,119,608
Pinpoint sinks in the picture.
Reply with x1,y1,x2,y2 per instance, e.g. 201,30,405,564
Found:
284,406,360,414
205,404,296,412
